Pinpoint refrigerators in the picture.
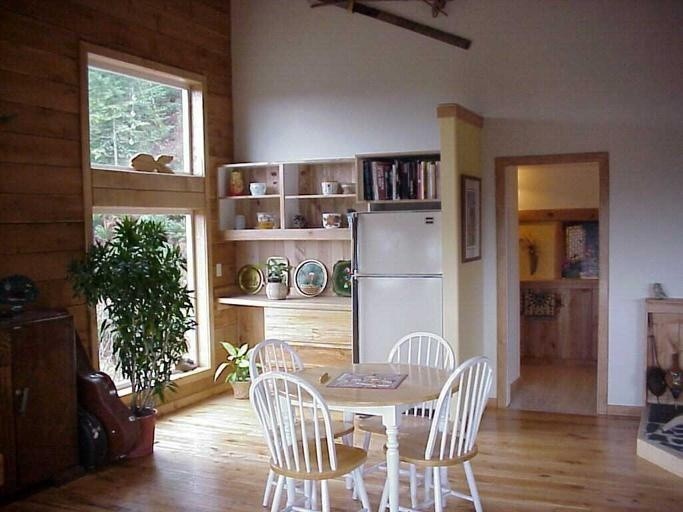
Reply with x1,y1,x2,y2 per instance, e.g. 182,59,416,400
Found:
351,210,444,362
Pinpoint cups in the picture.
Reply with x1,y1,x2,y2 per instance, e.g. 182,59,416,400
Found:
320,181,356,195
248,183,266,196
322,212,342,230
256,211,274,224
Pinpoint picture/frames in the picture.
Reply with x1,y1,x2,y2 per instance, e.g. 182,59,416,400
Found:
461,175,483,263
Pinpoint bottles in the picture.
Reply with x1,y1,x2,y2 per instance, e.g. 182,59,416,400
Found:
270,171,279,192
236,211,246,230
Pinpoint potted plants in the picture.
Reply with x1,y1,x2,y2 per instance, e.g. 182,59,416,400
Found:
63,215,199,460
214,340,265,400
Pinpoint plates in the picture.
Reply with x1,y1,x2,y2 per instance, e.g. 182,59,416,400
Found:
237,257,351,298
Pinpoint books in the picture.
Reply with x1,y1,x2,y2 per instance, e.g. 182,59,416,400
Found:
363,158,440,200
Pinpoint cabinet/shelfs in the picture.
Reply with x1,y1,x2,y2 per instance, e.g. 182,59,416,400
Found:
216,150,441,372
1,305,78,499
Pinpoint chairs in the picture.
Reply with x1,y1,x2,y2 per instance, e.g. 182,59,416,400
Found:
249,338,354,508
357,330,456,471
248,372,372,512
376,355,493,512
